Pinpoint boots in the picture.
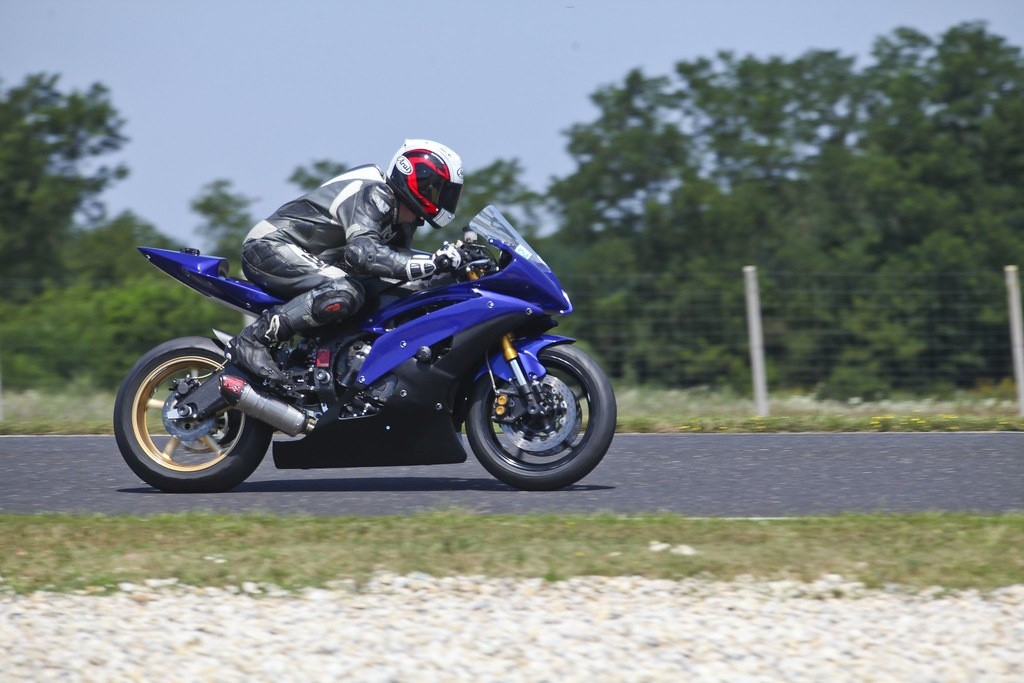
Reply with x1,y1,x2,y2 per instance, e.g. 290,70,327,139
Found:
225,300,294,382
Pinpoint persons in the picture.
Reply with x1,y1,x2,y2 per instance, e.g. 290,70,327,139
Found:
224,138,464,385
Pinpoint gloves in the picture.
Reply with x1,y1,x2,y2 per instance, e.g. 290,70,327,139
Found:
404,243,472,282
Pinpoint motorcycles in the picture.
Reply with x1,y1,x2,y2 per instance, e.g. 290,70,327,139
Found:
113,204,618,492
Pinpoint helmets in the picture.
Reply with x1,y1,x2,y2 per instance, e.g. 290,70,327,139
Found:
385,139,463,230
